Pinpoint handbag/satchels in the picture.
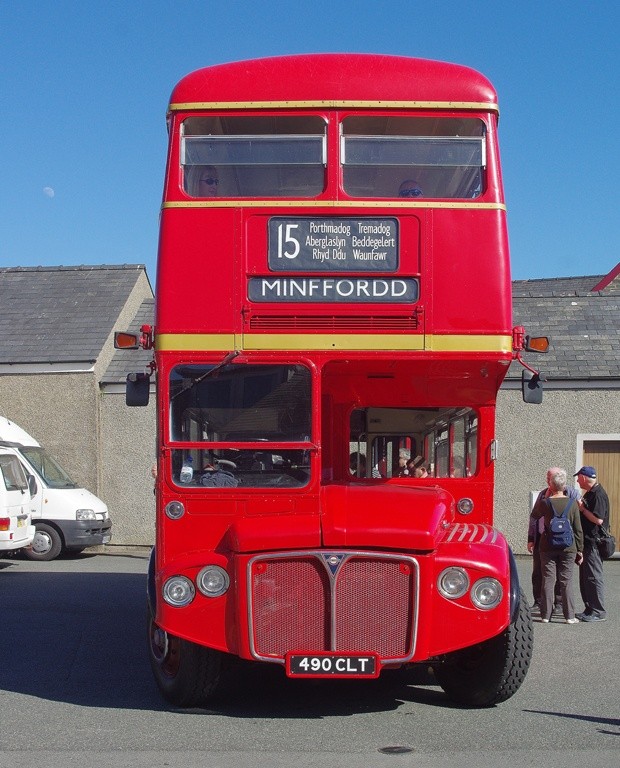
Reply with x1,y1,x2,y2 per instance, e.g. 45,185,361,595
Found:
597,536,615,558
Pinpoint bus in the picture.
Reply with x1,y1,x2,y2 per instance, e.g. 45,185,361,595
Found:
109,51,550,707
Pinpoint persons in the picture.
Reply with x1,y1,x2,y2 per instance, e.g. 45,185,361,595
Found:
398,180,423,197
526,466,611,624
187,164,219,197
350,445,473,480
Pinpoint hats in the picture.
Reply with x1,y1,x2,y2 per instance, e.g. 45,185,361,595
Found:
406,456,425,472
573,467,596,478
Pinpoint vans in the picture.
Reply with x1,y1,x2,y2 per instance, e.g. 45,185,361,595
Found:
0,446,37,554
0,415,112,561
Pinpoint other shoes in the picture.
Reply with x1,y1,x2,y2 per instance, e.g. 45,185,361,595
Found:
553,607,563,614
529,604,541,612
575,611,590,620
542,618,551,623
566,618,579,624
582,615,607,622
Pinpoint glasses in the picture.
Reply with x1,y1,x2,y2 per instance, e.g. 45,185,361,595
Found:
200,179,218,185
399,189,423,196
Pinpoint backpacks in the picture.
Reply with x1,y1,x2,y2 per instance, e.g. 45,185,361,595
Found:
546,498,573,549
